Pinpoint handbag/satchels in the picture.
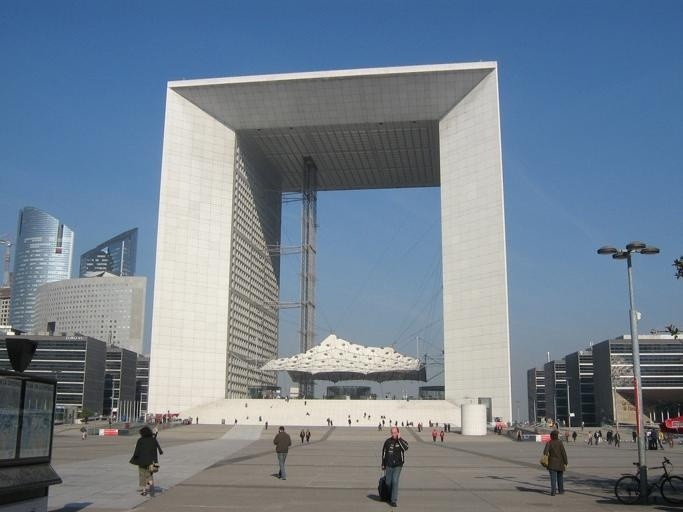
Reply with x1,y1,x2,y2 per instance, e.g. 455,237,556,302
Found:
540,455,549,466
149,463,159,473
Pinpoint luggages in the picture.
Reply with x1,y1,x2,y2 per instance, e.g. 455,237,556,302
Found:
378,468,387,501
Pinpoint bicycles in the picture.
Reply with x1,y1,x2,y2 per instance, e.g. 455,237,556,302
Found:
614,457,682,506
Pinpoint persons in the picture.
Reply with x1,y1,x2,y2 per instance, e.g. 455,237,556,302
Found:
646,427,674,450
299,428,306,443
129,425,164,496
274,426,291,480
542,429,568,497
632,430,637,443
494,424,503,434
234,394,311,429
80,426,87,439
586,429,622,447
517,429,522,442
196,416,199,424
325,412,451,443
380,425,408,507
572,431,577,442
306,428,311,445
565,430,570,442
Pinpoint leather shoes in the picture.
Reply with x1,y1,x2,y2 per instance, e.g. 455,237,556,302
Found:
140,485,154,496
278,474,286,480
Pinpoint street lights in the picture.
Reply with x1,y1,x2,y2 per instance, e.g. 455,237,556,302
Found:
598,242,660,499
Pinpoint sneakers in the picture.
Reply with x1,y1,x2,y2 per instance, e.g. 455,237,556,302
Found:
391,501,397,507
551,490,564,496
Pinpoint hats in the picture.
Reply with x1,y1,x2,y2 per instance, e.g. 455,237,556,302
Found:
279,426,285,429
